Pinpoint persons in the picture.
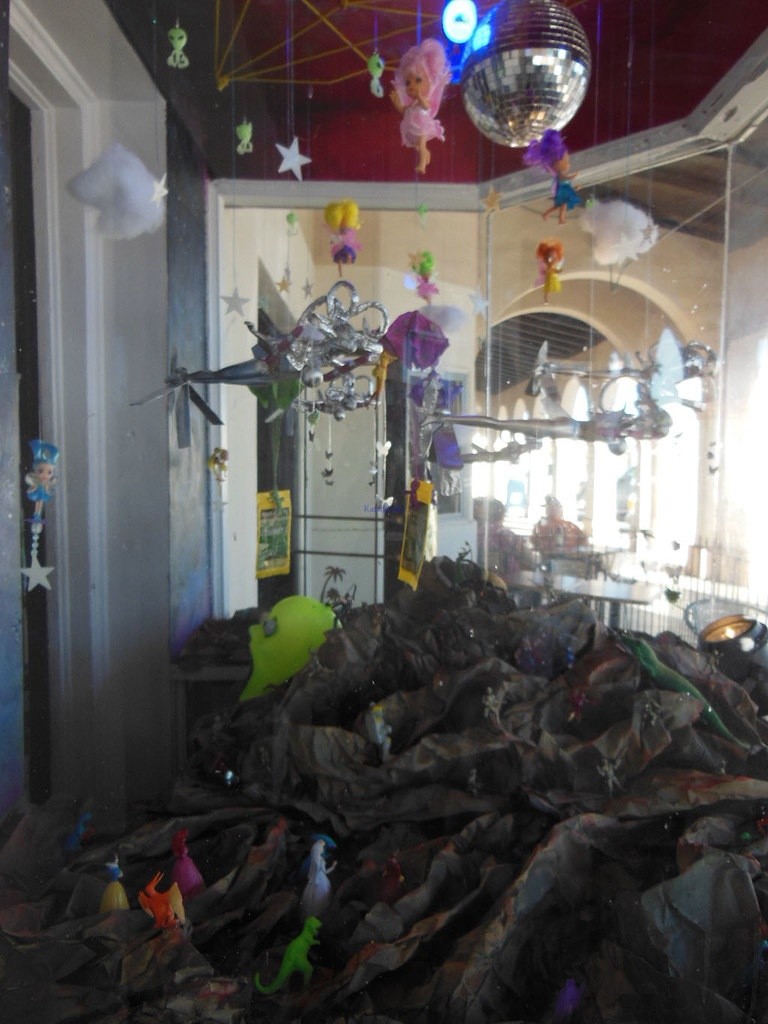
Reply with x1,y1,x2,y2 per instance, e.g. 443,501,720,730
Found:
529,495,637,585
299,839,338,921
99,850,130,914
170,829,207,903
522,129,583,226
325,196,363,277
408,249,440,305
536,239,565,304
389,37,452,174
24,440,60,522
473,495,538,574
366,706,395,765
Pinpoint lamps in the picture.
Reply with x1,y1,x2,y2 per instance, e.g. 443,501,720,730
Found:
697,613,768,716
